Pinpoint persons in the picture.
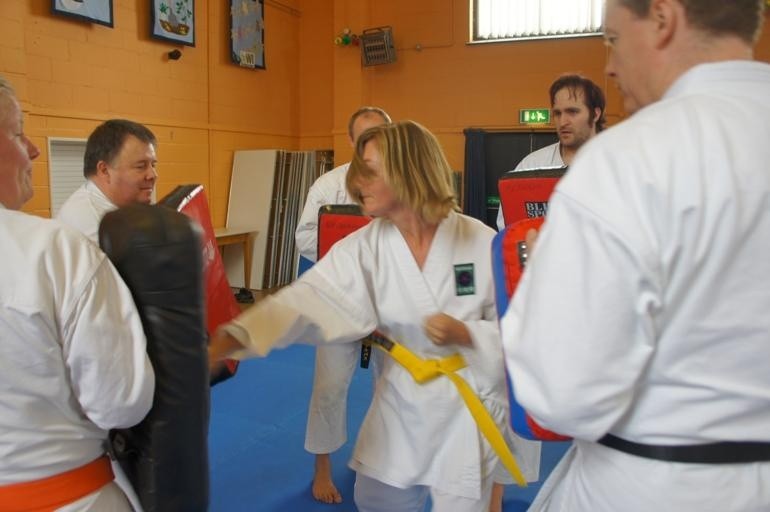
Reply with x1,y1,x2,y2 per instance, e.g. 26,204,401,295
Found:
53,117,158,248
294,105,394,505
208,118,502,512
0,80,159,512
496,72,607,232
499,0,770,512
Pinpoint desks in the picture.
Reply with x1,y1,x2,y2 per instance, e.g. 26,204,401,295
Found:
213,227,257,289
